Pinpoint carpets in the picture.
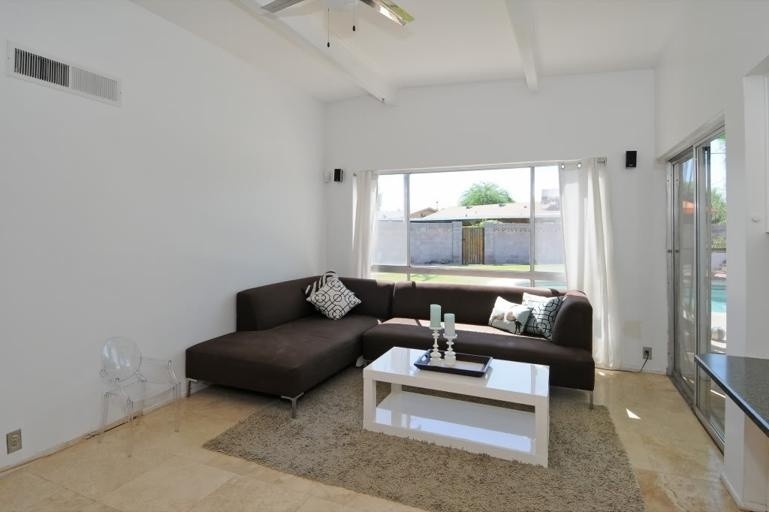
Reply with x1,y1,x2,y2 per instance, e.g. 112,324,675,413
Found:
202,366,645,512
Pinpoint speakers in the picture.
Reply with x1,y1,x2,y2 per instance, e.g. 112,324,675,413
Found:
334,169,343,182
626,151,637,167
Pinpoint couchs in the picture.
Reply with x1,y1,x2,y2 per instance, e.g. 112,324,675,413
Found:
362,283,595,408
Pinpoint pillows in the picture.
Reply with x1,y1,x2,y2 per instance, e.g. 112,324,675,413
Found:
488,291,567,341
300,270,361,320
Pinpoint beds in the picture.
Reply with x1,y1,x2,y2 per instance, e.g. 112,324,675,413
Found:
185,276,394,418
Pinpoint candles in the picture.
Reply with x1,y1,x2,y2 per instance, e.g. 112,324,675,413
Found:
430,304,441,327
444,313,455,337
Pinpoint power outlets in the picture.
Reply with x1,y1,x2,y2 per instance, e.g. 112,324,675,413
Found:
7,429,22,454
642,347,652,359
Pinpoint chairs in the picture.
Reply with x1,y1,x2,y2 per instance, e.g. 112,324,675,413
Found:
96,336,180,457
